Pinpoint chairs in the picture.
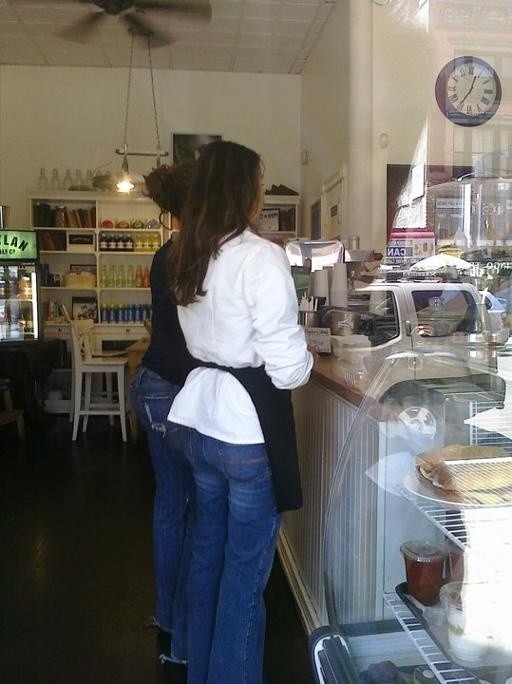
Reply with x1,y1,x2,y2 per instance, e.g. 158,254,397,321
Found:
58,317,129,442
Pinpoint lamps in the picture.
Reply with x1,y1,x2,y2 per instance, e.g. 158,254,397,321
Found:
116,28,169,193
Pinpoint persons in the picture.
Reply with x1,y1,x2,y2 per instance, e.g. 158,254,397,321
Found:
164,139,317,683
128,149,200,682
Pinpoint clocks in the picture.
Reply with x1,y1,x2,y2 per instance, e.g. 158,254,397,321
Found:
434,57,502,126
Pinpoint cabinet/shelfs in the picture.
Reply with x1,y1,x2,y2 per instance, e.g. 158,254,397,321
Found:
28,192,302,415
309,344,511,683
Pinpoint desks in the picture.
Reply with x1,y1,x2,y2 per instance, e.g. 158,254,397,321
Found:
125,337,151,445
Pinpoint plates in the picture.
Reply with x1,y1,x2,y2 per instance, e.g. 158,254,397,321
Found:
403,471,512,510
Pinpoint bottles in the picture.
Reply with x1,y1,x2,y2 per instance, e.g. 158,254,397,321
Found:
36,167,118,191
34,202,68,227
98,232,161,324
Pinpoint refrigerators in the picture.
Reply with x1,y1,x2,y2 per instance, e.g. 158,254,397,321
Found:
0,229,38,347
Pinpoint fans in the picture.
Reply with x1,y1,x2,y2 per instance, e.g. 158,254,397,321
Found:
52,1,212,49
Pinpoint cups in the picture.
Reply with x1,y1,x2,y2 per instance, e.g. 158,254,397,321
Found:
397,539,449,606
312,261,349,308
